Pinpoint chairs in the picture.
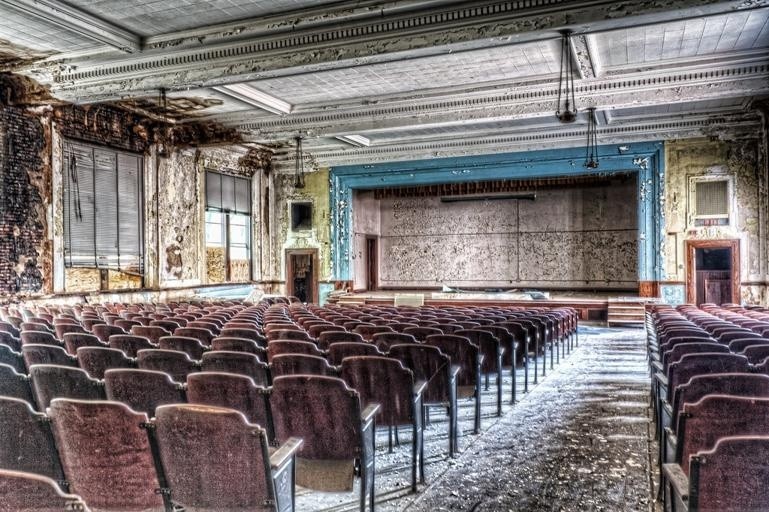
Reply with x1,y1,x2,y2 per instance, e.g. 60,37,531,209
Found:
644,303,769,512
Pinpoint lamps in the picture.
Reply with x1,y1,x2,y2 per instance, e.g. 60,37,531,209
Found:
585,107,599,168
155,87,172,158
556,29,577,123
294,136,306,188
